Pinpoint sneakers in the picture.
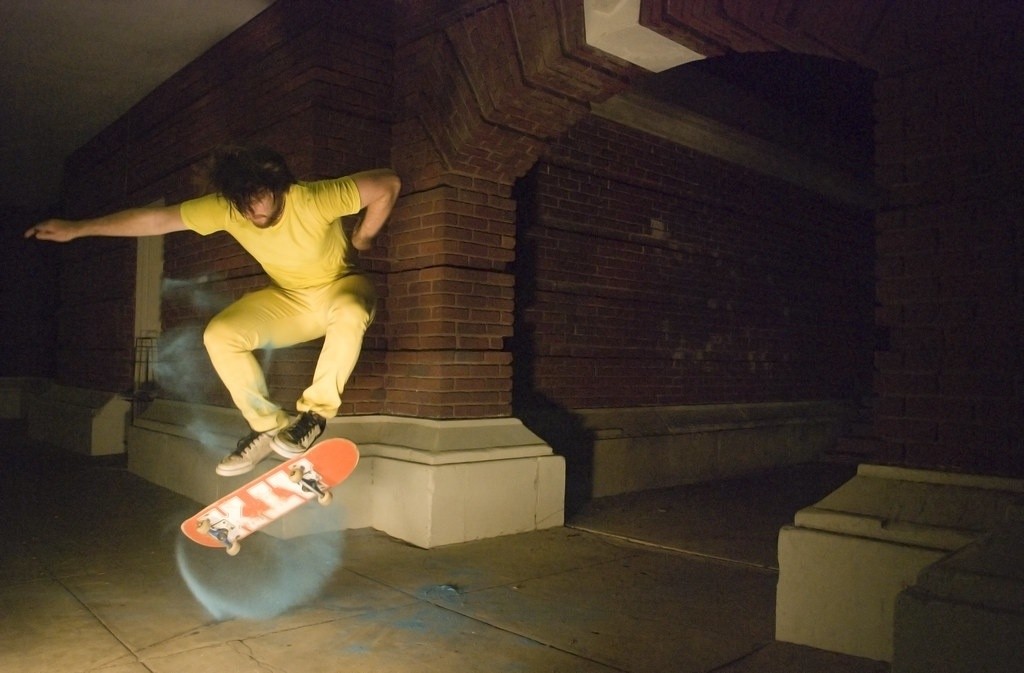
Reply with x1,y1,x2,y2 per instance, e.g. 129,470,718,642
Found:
270,410,325,457
215,413,292,477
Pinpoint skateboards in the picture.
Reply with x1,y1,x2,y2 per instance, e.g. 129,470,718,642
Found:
180,437,360,554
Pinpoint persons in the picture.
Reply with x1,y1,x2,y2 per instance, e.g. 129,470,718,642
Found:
22,146,401,478
